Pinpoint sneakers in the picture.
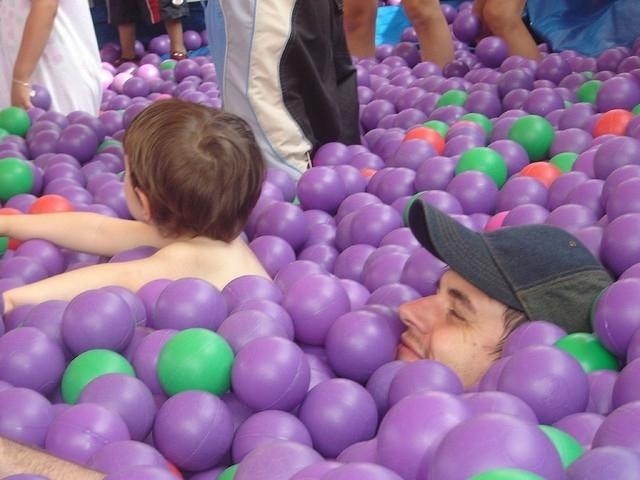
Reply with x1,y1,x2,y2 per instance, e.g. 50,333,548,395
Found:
113,55,140,66
172,51,187,59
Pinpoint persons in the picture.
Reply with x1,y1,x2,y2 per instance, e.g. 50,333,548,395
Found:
1,0,638,183
1,198,616,480
1,101,275,315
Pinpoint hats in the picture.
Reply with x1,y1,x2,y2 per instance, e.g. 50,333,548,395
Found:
409,199,613,334
